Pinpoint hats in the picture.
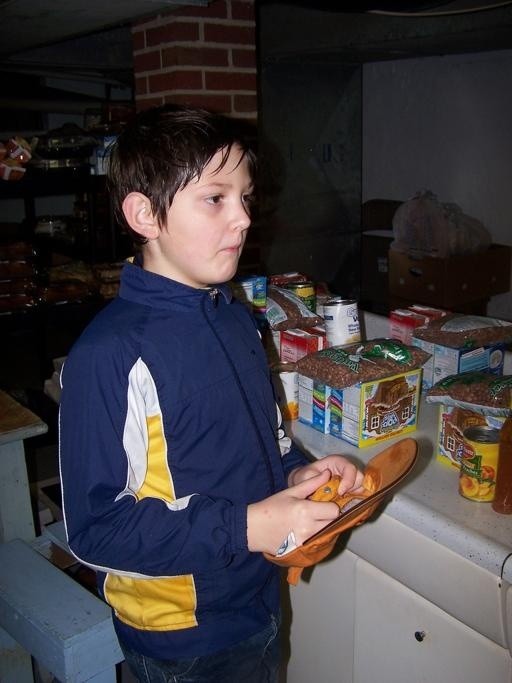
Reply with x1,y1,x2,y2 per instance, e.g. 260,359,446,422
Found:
262,436,419,585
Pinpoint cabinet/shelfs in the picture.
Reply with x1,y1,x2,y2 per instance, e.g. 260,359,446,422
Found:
388,243,512,326
261,90,365,282
286,513,512,682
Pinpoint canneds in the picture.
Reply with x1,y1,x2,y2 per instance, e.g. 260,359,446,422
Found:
271,361,300,421
285,283,363,349
458,425,500,505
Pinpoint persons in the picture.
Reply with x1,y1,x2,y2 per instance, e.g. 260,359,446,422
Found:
57,102,364,683
